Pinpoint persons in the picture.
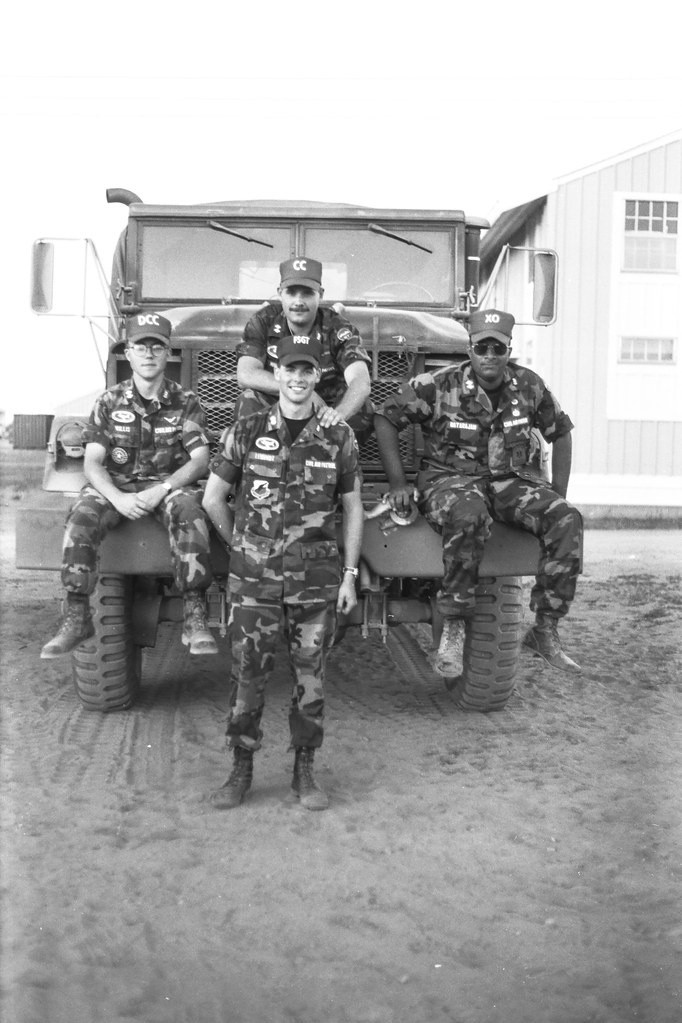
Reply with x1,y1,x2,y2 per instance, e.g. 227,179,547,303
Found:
199,334,366,811
39,311,221,661
370,309,585,680
232,255,373,427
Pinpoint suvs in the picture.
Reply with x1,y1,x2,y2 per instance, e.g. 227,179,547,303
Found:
32,188,558,713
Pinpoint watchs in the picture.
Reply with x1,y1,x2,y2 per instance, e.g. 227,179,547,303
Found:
342,566,359,579
160,480,173,494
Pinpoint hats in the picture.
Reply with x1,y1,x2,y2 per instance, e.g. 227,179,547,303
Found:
278,336,323,370
279,256,322,291
468,310,515,346
126,312,172,348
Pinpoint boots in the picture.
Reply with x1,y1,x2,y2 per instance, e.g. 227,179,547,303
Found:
526,614,582,676
434,619,467,679
40,594,95,659
210,745,254,809
291,744,328,809
182,597,219,655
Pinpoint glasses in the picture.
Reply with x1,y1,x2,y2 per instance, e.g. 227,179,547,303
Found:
468,343,507,356
126,341,167,357
